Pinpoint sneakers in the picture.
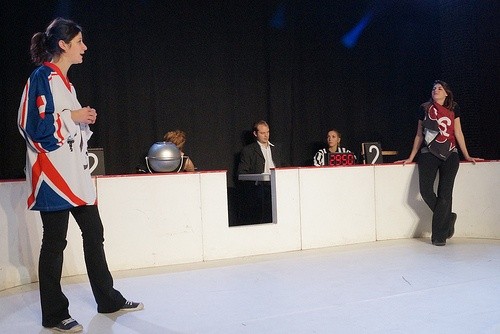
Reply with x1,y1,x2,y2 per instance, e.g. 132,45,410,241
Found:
96,299,144,313
45,316,84,333
448,212,457,239
432,238,448,247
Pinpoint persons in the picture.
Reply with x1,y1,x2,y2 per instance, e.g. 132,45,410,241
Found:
313,128,357,167
163,129,194,172
394,79,485,246
237,120,289,187
17,17,143,333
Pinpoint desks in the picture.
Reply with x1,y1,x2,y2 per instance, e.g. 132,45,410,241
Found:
238,174,271,222
